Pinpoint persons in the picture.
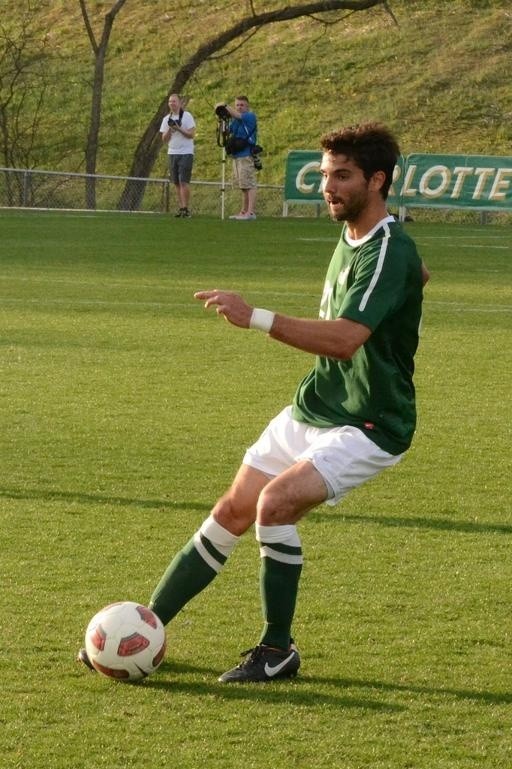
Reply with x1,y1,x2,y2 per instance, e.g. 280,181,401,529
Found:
214,94,258,221
158,92,197,218
74,121,431,682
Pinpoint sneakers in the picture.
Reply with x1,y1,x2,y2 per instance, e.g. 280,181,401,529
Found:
217,638,301,684
173,206,189,219
229,209,256,220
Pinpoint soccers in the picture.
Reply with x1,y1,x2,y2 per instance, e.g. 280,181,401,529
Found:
85,601,166,682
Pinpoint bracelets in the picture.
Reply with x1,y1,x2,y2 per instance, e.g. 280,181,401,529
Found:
246,307,275,333
224,104,227,108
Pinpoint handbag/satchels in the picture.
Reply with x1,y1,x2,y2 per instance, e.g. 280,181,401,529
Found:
224,127,257,155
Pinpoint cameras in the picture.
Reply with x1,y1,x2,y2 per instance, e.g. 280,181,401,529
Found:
216,106,230,119
167,118,183,128
